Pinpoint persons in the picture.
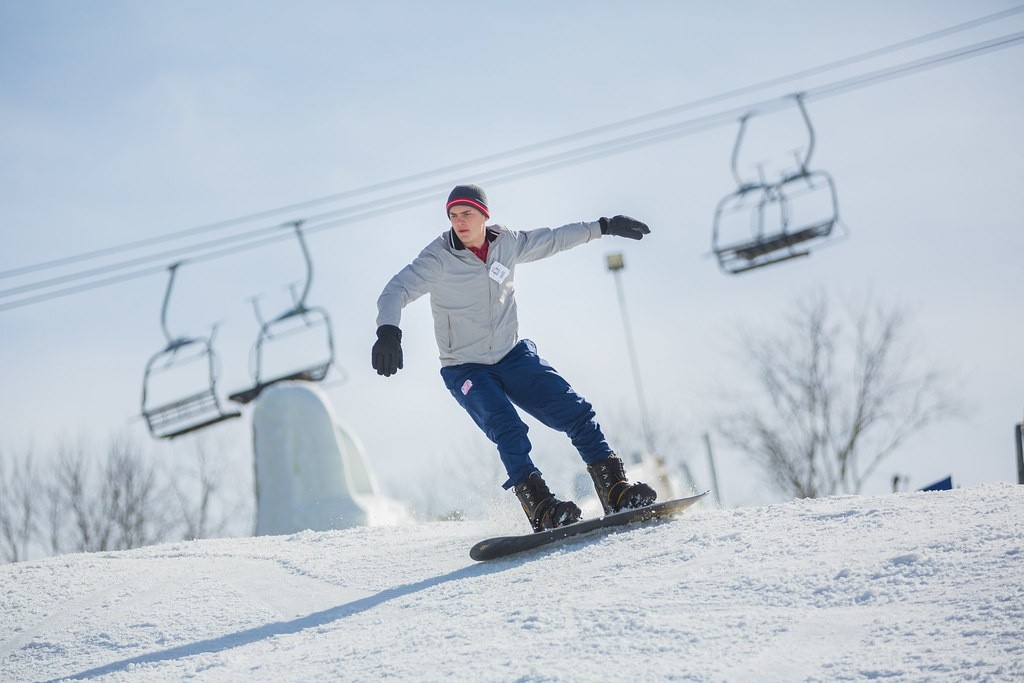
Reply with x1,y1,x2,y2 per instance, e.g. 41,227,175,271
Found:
371,184,656,534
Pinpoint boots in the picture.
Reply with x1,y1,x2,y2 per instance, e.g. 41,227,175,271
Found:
588,455,657,515
515,471,581,534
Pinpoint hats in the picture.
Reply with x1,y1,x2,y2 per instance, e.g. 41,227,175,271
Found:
446,184,490,220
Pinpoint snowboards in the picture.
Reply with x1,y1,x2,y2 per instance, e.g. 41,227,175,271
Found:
469,488,712,565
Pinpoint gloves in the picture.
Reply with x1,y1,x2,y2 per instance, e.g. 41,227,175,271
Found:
371,324,403,378
600,215,651,242
